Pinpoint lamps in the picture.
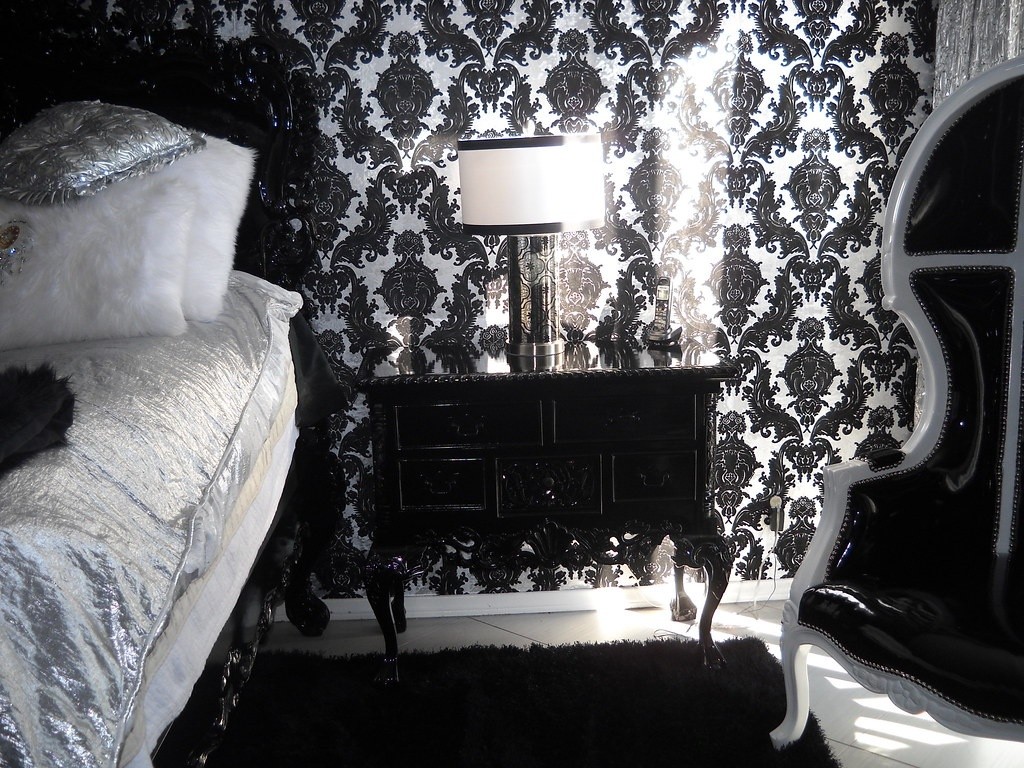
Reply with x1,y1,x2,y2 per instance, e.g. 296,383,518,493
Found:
463,131,603,371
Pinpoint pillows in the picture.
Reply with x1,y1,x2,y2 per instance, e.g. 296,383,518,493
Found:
0,100,206,205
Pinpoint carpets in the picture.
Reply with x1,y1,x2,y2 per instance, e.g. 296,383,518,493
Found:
162,636,839,768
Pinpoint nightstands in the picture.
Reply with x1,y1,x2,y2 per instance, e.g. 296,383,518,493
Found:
359,367,736,668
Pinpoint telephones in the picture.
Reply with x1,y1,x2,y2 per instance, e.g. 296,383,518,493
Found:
645,275,683,348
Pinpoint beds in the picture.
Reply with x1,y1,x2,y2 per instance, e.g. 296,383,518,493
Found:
0,272,317,768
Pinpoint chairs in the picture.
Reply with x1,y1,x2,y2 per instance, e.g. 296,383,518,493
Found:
771,54,1024,750
